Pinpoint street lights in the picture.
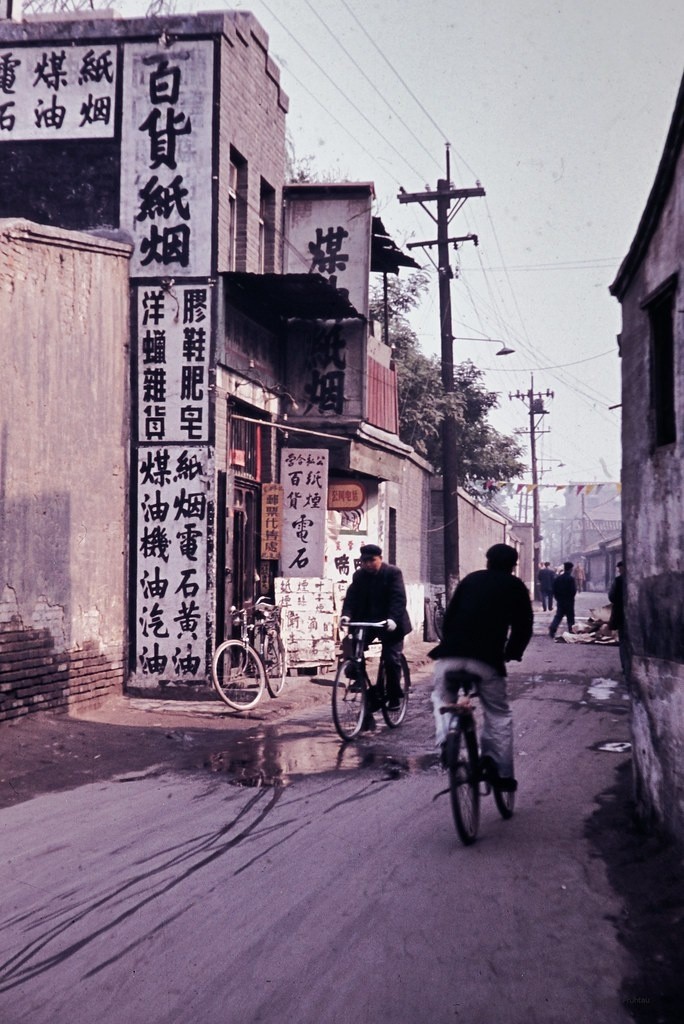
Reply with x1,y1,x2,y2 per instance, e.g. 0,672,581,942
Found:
439,335,517,607
532,458,567,602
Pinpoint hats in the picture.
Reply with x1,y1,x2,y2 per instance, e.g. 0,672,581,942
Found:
486,543,519,561
564,562,573,567
617,561,623,567
360,544,382,558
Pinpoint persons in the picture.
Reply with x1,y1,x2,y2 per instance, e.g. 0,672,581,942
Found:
574,562,586,593
427,544,534,793
337,544,413,712
539,562,555,611
549,562,577,639
608,561,625,673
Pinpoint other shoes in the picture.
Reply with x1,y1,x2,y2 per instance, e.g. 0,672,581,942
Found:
549,626,555,638
388,696,400,711
440,742,450,768
349,681,362,692
497,777,517,793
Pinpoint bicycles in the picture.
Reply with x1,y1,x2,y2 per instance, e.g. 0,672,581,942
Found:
331,618,412,741
431,669,518,845
211,594,289,711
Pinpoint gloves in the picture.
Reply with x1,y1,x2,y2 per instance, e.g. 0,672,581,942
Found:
383,619,397,632
337,616,350,631
